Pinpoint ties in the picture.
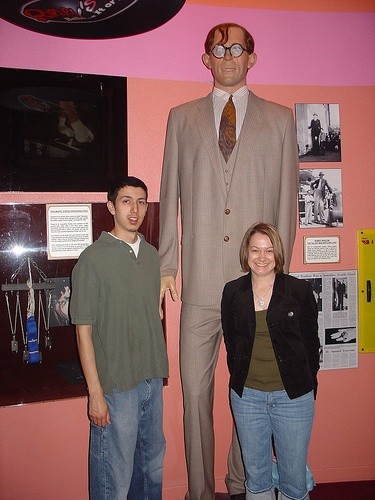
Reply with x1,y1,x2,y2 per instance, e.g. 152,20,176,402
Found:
218,95,237,163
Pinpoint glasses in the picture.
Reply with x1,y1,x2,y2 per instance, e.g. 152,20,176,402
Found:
207,43,248,59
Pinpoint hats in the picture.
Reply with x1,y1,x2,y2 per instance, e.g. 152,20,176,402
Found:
319,172,325,176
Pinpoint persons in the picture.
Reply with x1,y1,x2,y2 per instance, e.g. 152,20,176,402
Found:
305,190,314,226
71,177,169,500
312,171,333,224
159,23,296,500
221,225,321,500
307,114,323,154
337,280,346,311
